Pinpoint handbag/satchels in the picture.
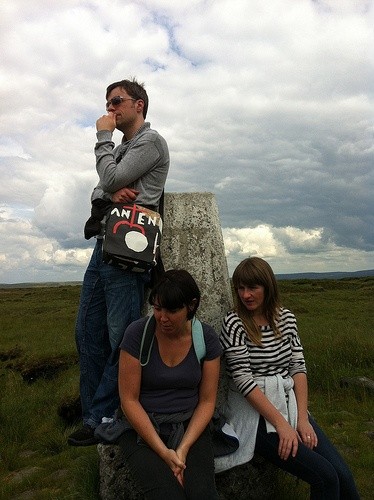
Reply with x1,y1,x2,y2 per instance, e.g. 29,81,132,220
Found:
102,204,162,269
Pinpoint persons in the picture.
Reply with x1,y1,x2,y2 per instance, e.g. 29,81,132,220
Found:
67,78,170,445
93,268,221,500
218,257,361,500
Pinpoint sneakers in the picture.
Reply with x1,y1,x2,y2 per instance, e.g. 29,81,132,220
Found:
67,425,100,446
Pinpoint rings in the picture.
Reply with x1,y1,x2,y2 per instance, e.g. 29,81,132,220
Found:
306,436,311,439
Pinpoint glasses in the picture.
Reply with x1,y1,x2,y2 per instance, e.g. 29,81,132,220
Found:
106,95,138,108
